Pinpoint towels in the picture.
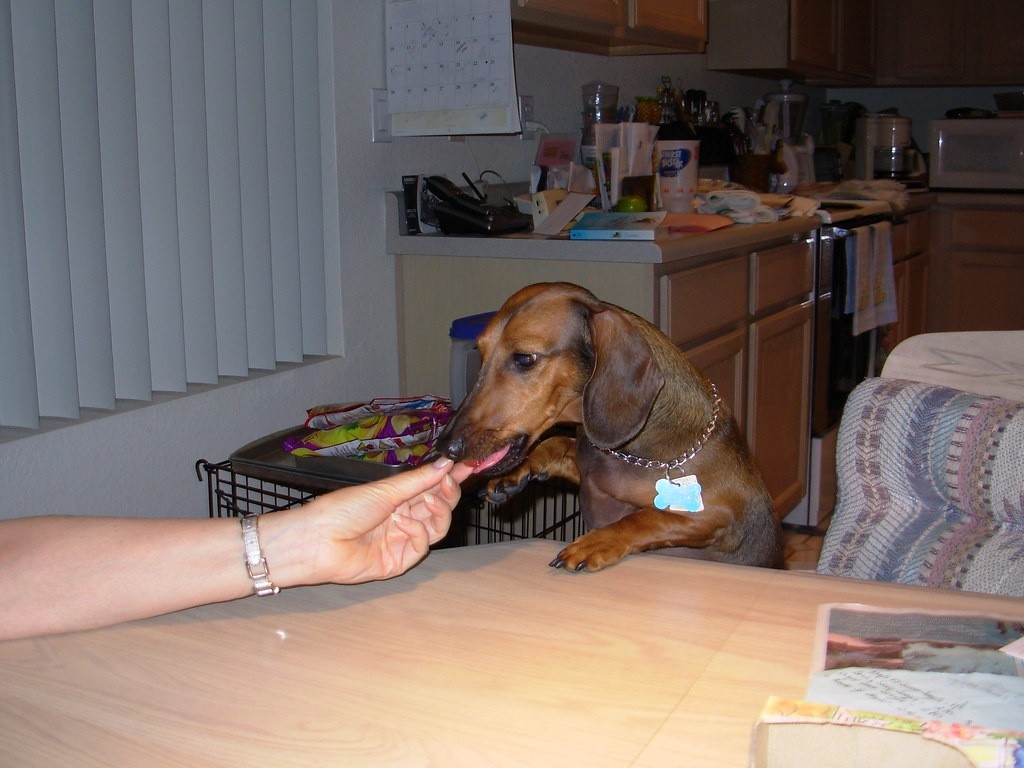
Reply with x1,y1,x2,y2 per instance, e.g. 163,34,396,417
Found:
843,220,898,336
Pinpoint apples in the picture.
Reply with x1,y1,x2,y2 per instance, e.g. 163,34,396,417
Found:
615,196,646,212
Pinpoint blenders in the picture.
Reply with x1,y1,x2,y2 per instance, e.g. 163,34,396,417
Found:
766,90,814,185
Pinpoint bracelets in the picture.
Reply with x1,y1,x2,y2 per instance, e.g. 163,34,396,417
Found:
241,514,274,596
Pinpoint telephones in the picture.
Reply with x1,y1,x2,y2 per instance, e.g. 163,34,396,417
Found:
423,175,528,235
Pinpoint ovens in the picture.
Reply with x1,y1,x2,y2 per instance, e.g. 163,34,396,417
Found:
805,218,908,533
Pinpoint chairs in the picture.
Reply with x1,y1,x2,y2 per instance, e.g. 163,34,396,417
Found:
816,375,1024,603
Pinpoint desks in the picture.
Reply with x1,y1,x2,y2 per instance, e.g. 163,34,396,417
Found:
0,535,1023,768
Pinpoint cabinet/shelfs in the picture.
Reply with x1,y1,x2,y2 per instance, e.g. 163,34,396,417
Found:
397,208,1024,530
502,0,1024,88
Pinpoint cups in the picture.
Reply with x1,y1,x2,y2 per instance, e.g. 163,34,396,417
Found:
656,140,701,213
581,84,619,145
689,100,720,128
854,115,912,185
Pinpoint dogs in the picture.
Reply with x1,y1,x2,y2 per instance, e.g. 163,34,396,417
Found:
435,280,786,571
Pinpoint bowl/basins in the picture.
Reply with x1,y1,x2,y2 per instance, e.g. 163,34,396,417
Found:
993,91,1024,112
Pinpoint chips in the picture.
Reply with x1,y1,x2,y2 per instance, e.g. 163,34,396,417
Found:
392,414,429,461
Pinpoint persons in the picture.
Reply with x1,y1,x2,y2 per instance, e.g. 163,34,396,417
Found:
0,456,476,640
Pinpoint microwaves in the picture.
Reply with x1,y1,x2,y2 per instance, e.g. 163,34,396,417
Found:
929,117,1024,190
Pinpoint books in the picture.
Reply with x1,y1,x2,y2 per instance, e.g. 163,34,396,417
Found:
570,209,670,241
595,121,661,209
